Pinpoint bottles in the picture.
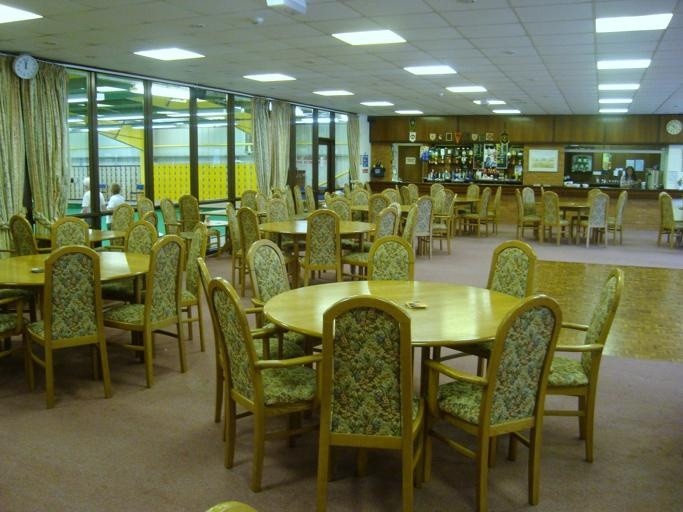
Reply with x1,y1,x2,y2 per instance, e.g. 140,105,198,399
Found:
427,148,472,166
430,168,508,182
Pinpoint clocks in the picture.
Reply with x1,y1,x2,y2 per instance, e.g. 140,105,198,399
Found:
13,55,38,79
665,120,682,136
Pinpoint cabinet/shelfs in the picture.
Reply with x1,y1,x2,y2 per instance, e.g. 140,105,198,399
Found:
424,145,524,184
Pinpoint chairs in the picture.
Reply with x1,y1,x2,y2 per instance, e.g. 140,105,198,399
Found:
657,191,683,247
433,239,537,378
508,267,625,506
197,255,322,493
226,181,502,298
515,185,628,249
161,195,230,264
315,294,425,512
23,244,114,411
246,239,320,347
0,197,208,391
425,294,564,512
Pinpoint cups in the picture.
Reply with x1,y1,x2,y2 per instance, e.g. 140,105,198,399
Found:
640,185,646,189
640,182,646,185
582,184,589,188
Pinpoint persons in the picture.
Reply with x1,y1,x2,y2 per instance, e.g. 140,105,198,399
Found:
105,183,126,230
620,166,641,187
82,178,106,229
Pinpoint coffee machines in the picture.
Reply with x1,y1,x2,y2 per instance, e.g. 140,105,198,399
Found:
591,169,625,187
